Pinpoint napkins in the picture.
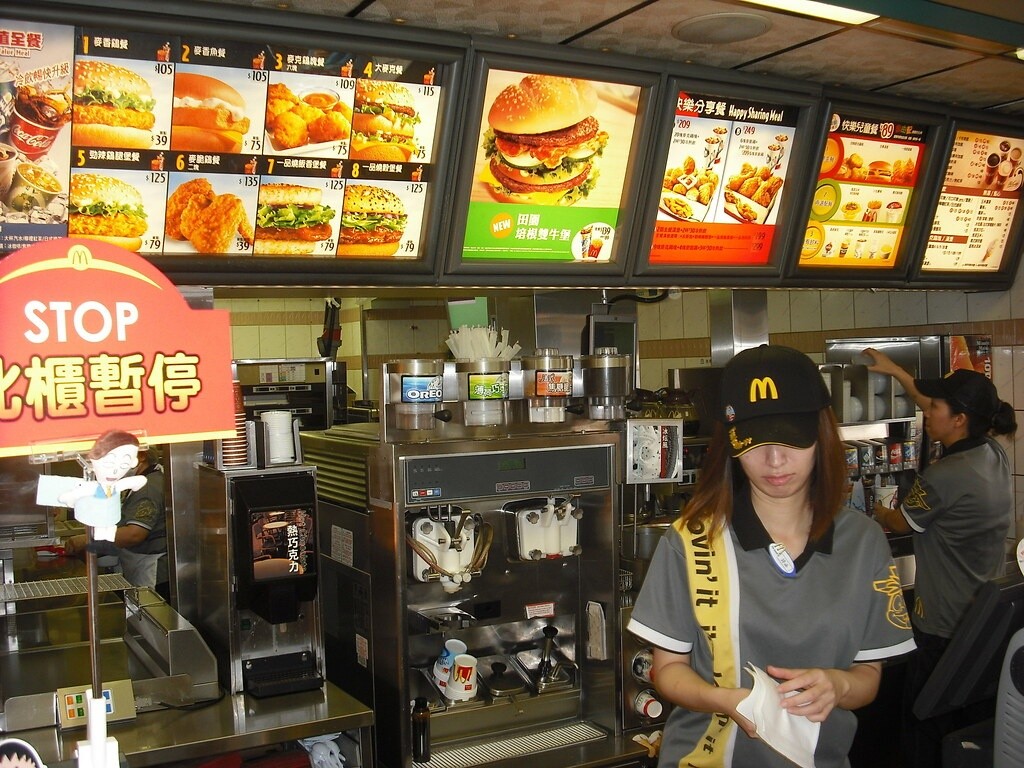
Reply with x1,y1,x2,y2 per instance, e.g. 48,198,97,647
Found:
735,661,821,767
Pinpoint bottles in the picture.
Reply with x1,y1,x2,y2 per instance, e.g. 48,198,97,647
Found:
411,698,431,762
636,694,662,718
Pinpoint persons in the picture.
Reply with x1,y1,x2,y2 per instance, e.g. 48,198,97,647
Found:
861,347,1017,768
64,430,172,606
626,344,918,768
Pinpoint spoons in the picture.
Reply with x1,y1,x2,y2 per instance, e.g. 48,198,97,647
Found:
310,740,346,768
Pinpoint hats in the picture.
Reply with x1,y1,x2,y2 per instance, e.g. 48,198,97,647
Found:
913,368,1000,419
716,343,831,459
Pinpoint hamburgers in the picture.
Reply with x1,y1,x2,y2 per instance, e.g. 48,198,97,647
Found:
70,175,147,251
338,184,407,255
172,72,249,154
350,80,418,162
867,161,892,182
486,72,598,205
254,184,335,254
74,62,155,149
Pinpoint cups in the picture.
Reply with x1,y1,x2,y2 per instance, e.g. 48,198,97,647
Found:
581,233,592,259
5,163,62,213
984,153,1001,185
887,208,903,221
0,82,16,141
0,142,17,202
433,639,479,699
999,140,1010,162
881,244,893,259
869,245,878,258
895,555,917,585
1010,147,1022,166
855,239,866,258
222,379,249,467
875,485,898,510
997,160,1012,190
9,97,65,162
